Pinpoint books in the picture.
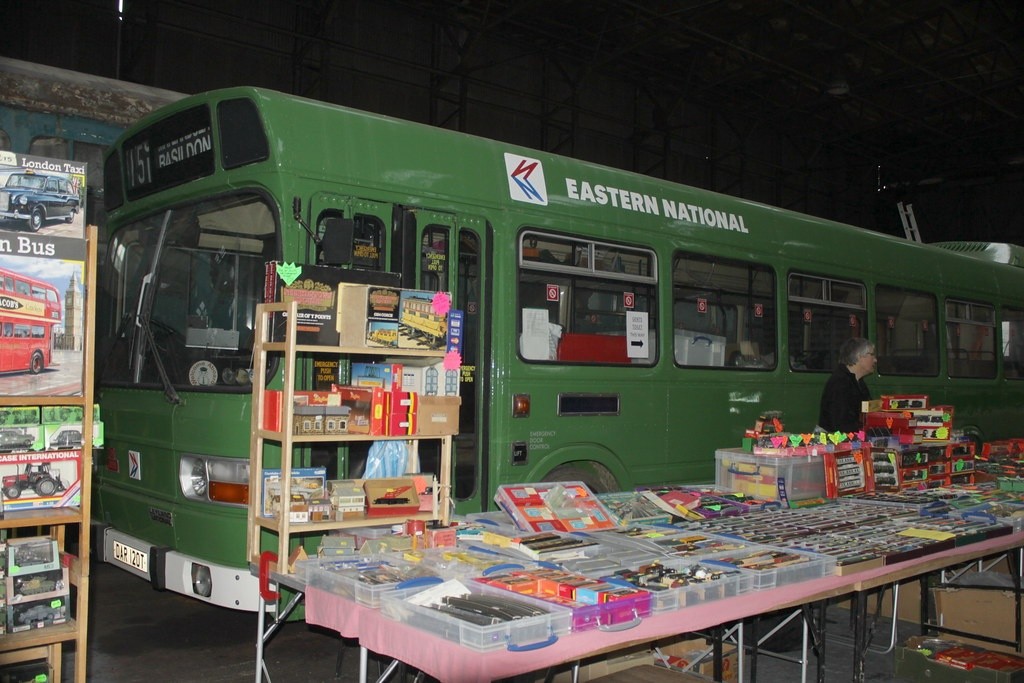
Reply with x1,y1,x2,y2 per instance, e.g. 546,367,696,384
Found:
935,645,1024,672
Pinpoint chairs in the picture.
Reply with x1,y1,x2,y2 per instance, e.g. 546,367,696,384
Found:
796,347,997,376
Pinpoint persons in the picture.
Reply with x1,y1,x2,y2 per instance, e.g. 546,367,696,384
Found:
818,336,878,434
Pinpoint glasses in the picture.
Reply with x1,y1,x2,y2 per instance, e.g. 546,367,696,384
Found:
866,352,874,356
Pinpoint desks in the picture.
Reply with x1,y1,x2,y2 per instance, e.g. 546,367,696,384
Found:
251,530,1024,683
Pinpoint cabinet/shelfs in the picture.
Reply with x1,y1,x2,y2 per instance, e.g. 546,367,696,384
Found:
0,150,98,683
246,281,462,576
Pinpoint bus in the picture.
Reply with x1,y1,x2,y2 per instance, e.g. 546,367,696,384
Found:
90,87,1023,624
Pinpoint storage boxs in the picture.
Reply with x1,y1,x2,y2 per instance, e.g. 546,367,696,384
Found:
893,636,1024,683
714,447,827,501
262,384,461,522
513,631,745,683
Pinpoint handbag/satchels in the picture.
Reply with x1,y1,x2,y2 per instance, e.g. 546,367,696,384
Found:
707,608,811,653
